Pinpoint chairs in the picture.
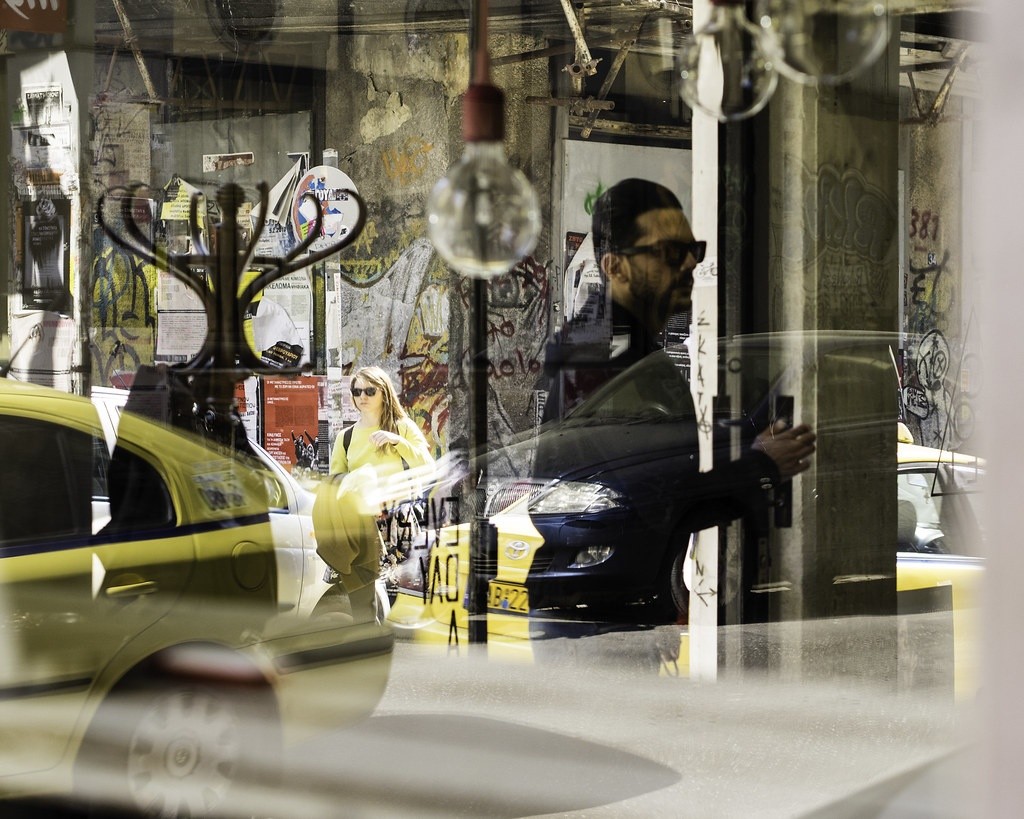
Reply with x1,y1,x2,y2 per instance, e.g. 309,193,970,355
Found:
898,501,918,553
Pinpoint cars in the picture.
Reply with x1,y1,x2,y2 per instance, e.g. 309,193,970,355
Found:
358,328,937,650
1,376,399,819
416,423,986,660
90,383,390,625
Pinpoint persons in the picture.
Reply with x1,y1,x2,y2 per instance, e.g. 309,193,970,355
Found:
524,177,816,640
330,367,434,603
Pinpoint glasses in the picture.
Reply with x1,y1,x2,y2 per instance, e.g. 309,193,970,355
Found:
350,387,377,397
617,237,706,266
